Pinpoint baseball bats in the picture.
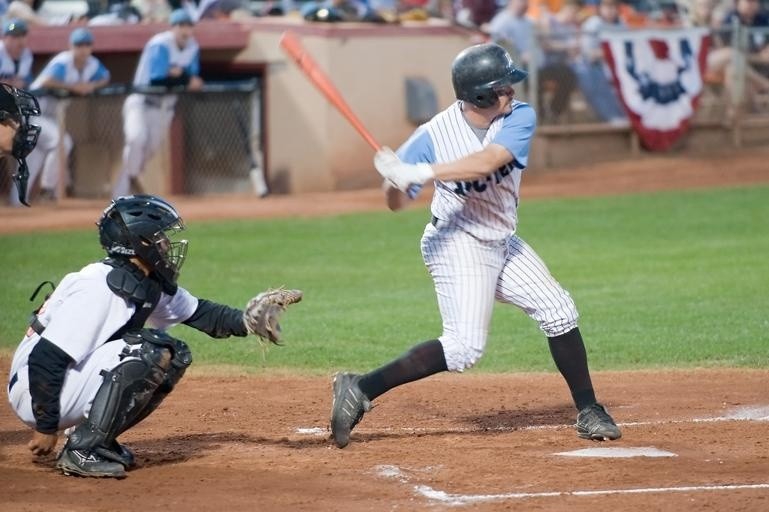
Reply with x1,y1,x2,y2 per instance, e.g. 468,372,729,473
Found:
280,31,424,200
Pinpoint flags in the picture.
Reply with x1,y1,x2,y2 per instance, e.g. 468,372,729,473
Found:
600,34,710,153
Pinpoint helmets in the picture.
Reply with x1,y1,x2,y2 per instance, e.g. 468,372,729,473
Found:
0,82,42,161
95,193,190,296
450,42,529,110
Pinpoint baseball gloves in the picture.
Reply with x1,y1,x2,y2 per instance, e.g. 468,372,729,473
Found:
242,288,302,348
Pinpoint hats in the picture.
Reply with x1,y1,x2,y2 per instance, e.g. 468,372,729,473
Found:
3,8,195,46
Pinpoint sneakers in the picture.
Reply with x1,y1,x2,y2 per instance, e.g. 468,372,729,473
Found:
330,371,379,450
537,87,769,126
575,403,622,440
52,434,135,477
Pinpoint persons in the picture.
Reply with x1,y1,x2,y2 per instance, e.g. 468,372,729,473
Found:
7,25,110,203
6,193,303,478
114,9,202,197
0,80,40,208
330,44,622,449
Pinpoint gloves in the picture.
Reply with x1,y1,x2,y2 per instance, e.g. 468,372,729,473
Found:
373,145,434,195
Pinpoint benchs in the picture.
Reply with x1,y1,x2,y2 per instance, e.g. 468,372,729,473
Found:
529,67,768,166
